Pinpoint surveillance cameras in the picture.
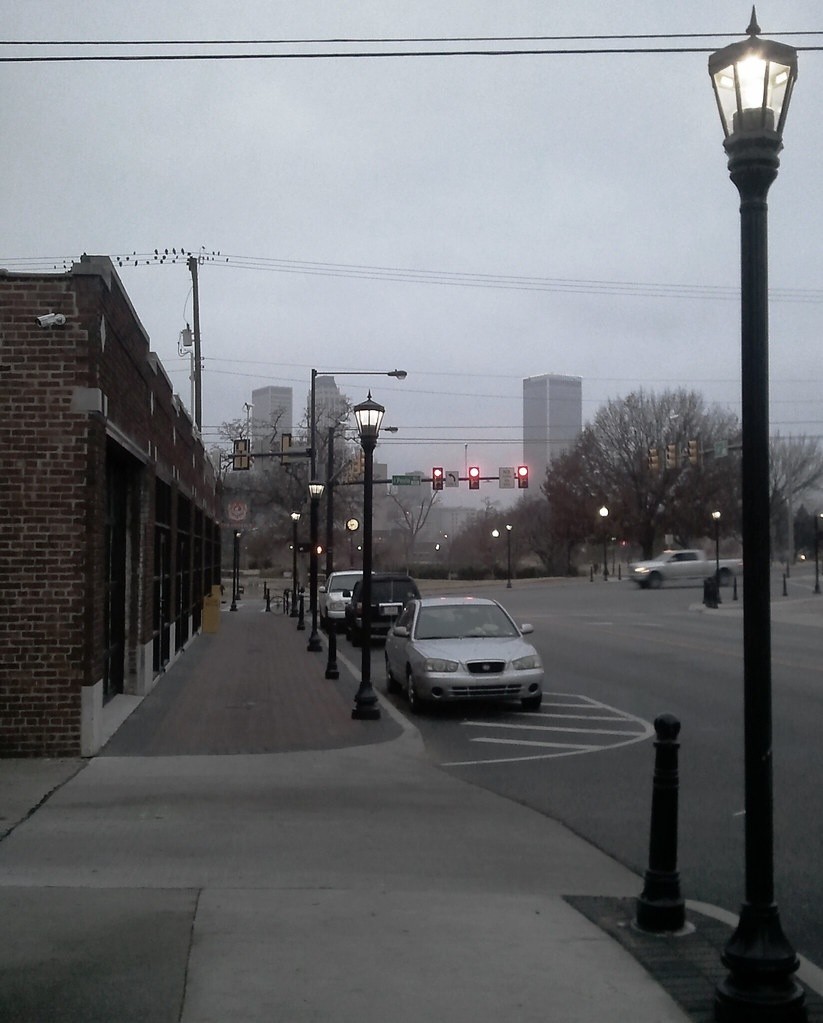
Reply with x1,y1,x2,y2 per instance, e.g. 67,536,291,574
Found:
35,314,55,327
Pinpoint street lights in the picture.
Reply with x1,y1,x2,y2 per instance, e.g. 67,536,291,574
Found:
705,2,810,1023
326,426,399,579
600,506,608,581
307,479,327,654
306,368,408,613
351,389,382,721
712,508,722,604
289,509,301,618
506,524,512,587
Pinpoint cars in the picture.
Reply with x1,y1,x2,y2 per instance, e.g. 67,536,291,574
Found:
385,598,544,716
343,573,423,646
319,570,375,632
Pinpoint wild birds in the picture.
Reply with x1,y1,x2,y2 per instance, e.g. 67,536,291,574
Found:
54,252,87,269
117,246,229,267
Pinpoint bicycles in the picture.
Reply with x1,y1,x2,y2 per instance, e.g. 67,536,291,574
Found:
269,589,310,615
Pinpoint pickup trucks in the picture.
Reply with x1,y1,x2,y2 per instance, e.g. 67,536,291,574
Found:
629,549,744,591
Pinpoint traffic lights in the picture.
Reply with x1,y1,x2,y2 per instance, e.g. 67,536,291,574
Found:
468,467,479,490
518,466,528,489
648,449,658,471
667,445,676,470
687,441,697,465
432,467,443,491
